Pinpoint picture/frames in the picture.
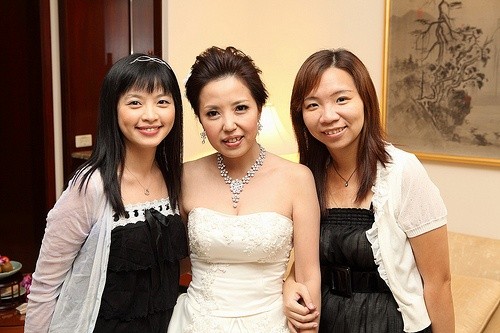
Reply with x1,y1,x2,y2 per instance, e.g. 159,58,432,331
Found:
380,0,500,168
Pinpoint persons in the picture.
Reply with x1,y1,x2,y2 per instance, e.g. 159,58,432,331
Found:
23,52,190,333
160,45,323,333
281,48,455,333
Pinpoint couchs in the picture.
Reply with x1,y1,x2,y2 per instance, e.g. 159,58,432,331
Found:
447,231,500,333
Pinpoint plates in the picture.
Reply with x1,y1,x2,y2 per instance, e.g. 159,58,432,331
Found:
0,260,23,279
0,285,27,299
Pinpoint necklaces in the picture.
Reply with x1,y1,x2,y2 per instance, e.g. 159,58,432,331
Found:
331,162,357,188
216,143,266,210
124,161,157,196
